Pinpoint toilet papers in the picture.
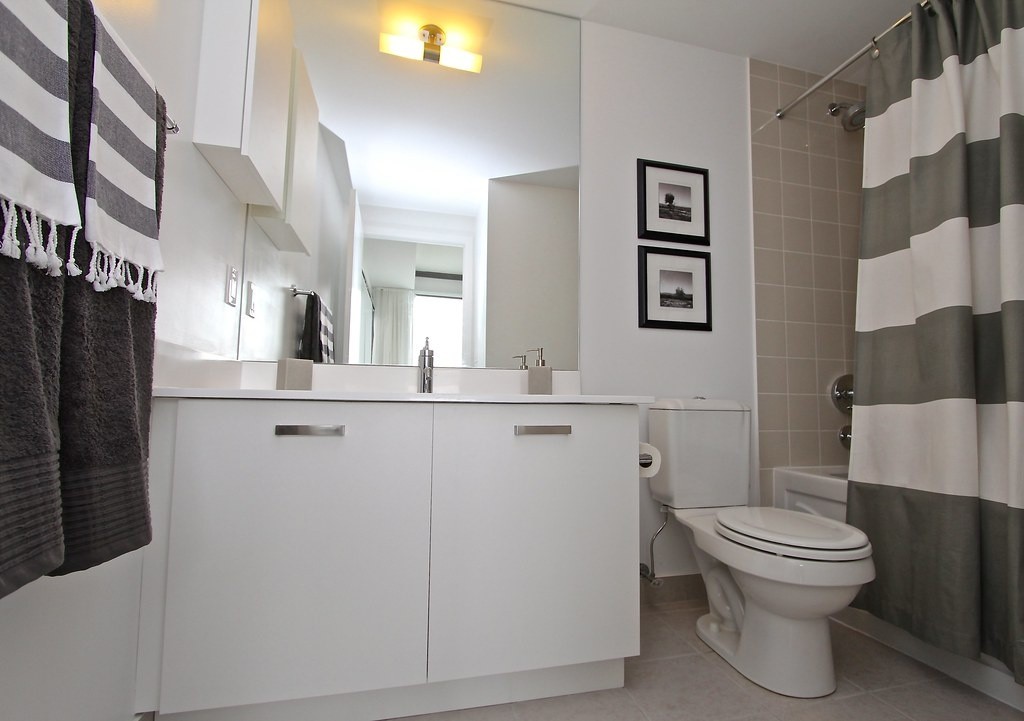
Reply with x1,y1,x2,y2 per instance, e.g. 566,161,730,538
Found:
637,442,664,478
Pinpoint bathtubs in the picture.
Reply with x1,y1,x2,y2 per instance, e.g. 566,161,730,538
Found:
764,457,1024,719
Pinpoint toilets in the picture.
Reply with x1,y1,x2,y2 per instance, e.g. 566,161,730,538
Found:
649,396,880,702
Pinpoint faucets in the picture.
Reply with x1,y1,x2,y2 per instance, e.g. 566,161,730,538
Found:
417,337,436,394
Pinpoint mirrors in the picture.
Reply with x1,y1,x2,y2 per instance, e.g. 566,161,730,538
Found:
237,0,584,373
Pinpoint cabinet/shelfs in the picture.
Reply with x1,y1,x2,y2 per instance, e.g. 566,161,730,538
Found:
251,48,320,255
190,0,294,213
138,386,650,721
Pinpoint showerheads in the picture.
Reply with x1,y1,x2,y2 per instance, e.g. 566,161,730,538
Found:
840,101,867,135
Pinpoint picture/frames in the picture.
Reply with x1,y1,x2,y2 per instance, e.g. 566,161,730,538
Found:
636,245,714,333
636,157,712,246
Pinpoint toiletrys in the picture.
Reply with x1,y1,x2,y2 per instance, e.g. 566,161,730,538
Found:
512,355,531,370
523,347,553,396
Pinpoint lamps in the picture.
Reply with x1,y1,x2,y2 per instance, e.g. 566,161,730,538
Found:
379,25,484,74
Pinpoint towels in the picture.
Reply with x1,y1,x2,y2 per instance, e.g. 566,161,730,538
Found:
0,0,89,282
0,0,80,604
81,9,169,304
75,4,169,579
297,293,335,364
